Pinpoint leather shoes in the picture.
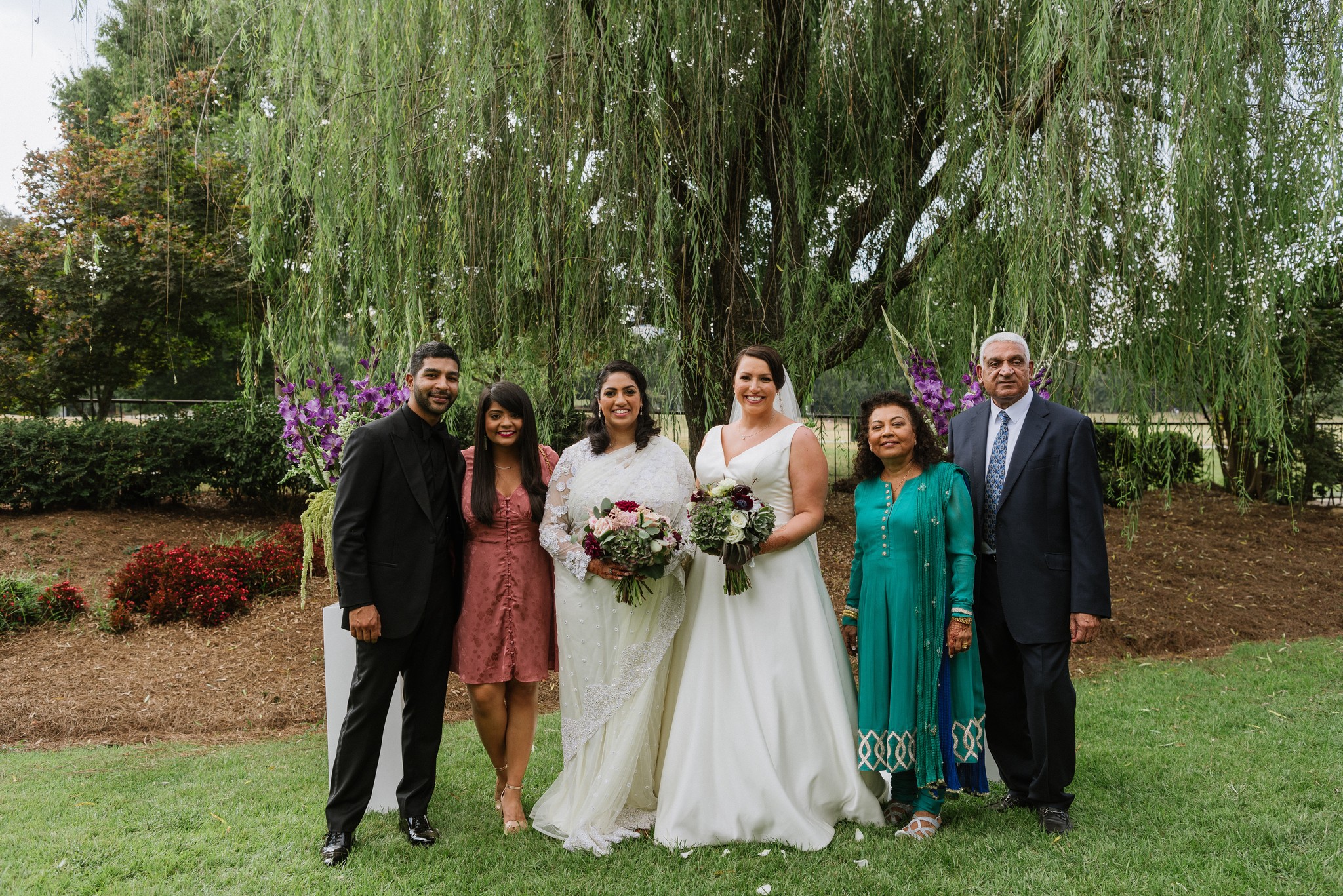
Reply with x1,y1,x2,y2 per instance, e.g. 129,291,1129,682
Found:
983,794,1033,814
1038,806,1072,835
400,814,437,846
319,830,350,866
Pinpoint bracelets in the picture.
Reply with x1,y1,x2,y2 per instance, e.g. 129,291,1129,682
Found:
952,617,973,625
842,605,859,620
951,608,972,616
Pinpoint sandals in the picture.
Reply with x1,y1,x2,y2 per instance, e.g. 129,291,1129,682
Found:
883,801,912,826
896,814,942,841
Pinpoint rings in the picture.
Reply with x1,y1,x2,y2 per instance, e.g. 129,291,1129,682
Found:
609,568,612,574
962,643,968,649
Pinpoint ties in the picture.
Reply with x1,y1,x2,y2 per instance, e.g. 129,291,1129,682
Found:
982,411,1010,551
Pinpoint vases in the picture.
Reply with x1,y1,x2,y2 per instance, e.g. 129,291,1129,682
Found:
321,602,407,815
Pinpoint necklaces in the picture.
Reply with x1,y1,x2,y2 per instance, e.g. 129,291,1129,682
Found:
494,462,521,469
883,461,915,498
739,409,775,440
609,444,616,451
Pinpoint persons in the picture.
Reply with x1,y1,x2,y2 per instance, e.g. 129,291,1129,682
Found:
946,332,1111,837
449,380,559,835
320,340,468,868
841,390,989,842
651,345,891,852
529,361,696,857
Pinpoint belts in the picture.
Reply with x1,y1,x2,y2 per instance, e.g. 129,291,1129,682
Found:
979,552,998,563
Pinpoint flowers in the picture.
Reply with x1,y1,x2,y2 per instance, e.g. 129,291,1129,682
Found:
884,285,1069,456
686,478,776,599
273,348,412,614
580,496,684,611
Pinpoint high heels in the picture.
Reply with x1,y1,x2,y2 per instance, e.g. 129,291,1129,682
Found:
494,763,508,810
499,783,529,837
